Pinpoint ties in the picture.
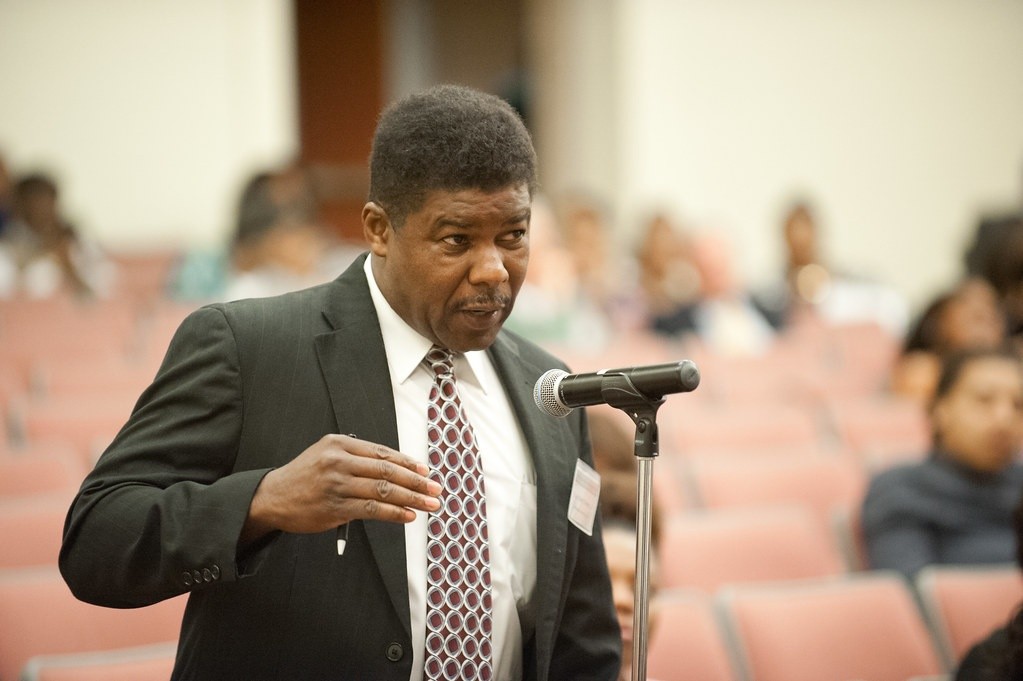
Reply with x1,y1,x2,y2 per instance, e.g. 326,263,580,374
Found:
423,345,493,681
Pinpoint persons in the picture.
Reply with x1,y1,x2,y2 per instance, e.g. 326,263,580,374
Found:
857,207,1022,681
582,411,666,681
0,154,103,302
222,170,837,349
58,86,623,681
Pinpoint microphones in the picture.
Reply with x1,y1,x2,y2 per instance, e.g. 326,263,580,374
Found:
533,360,701,417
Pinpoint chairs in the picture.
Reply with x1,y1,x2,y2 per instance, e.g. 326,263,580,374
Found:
0,249,1023,681
